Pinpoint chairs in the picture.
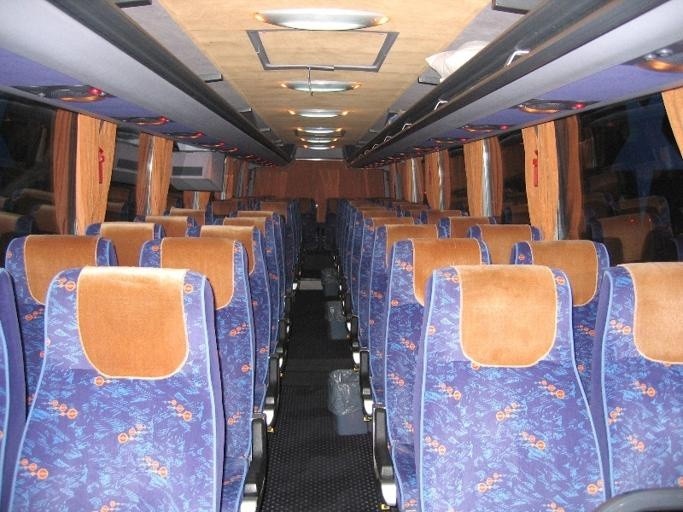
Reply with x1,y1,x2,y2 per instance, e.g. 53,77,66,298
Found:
371,239,492,511
9,266,224,511
414,264,607,508
592,263,682,508
1,196,645,417
137,238,266,511
0,268,30,511
